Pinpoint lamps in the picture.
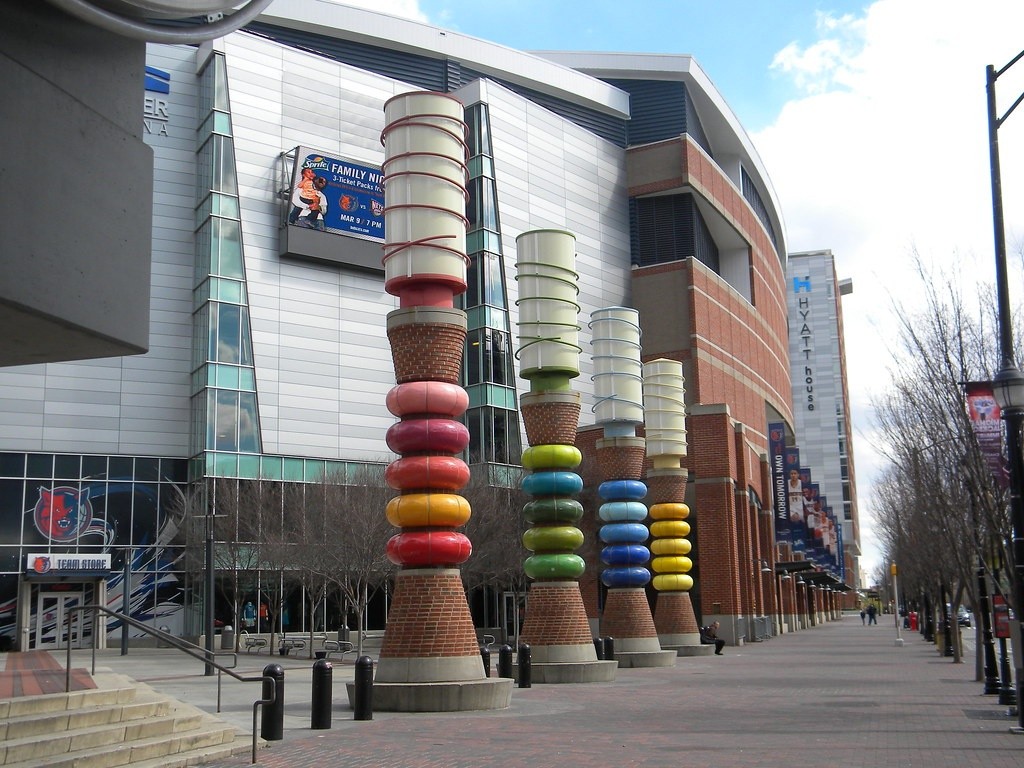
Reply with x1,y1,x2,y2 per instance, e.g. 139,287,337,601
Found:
818,584,846,594
796,575,804,584
808,580,815,587
782,569,790,579
760,560,771,572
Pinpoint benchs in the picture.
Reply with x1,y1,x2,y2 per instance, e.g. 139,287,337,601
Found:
277,639,306,656
244,637,266,654
322,639,354,662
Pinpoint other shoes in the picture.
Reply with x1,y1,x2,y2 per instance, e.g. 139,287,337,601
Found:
715,652,723,655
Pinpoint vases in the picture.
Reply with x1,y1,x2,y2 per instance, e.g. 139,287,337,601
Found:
314,652,325,660
278,648,289,656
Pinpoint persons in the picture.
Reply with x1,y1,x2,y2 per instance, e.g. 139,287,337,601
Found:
259,602,268,633
860,609,866,624
244,601,256,633
813,500,822,529
821,509,830,553
786,468,803,523
866,603,877,625
834,530,839,566
702,620,725,655
829,519,836,557
291,168,327,231
801,486,814,542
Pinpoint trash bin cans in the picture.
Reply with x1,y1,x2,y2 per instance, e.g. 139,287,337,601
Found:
221,625,234,649
338,624,349,650
937,620,964,657
158,625,170,648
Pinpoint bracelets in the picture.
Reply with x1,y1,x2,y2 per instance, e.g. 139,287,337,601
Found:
266,616,268,617
254,616,255,618
